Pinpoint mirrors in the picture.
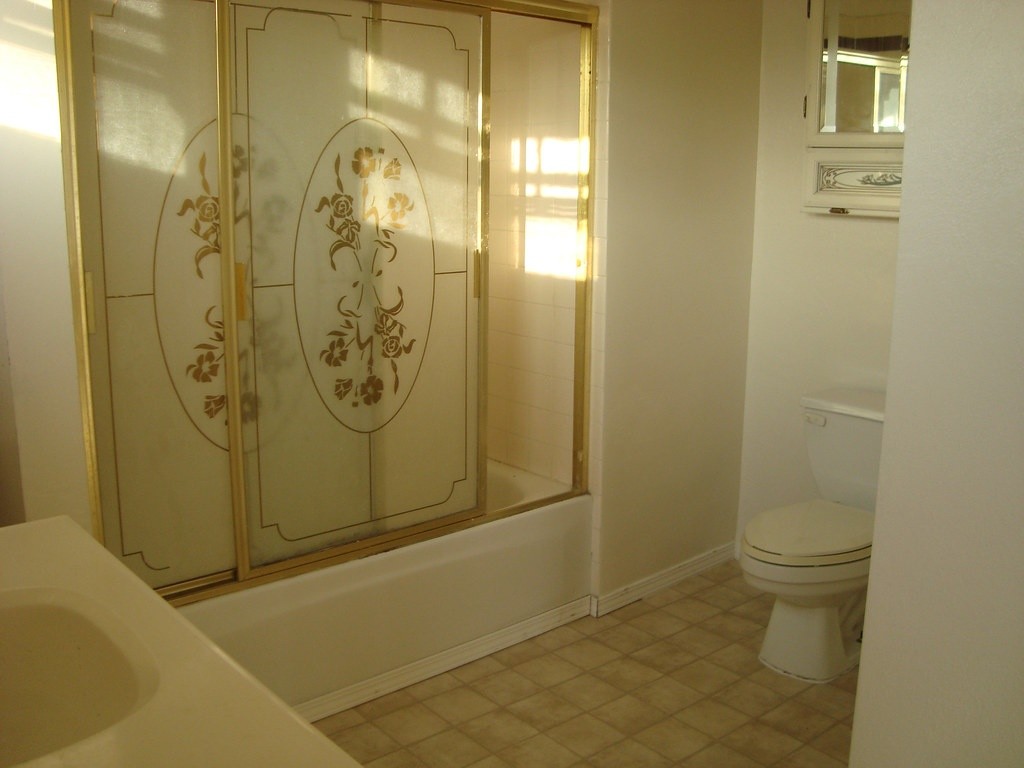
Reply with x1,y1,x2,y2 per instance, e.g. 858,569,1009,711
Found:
803,0,908,149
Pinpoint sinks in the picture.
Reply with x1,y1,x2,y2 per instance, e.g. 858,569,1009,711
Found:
0,585,159,768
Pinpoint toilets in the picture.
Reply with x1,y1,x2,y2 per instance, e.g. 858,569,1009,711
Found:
739,385,885,684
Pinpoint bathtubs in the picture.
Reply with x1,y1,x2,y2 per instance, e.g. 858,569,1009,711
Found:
172,448,592,727
